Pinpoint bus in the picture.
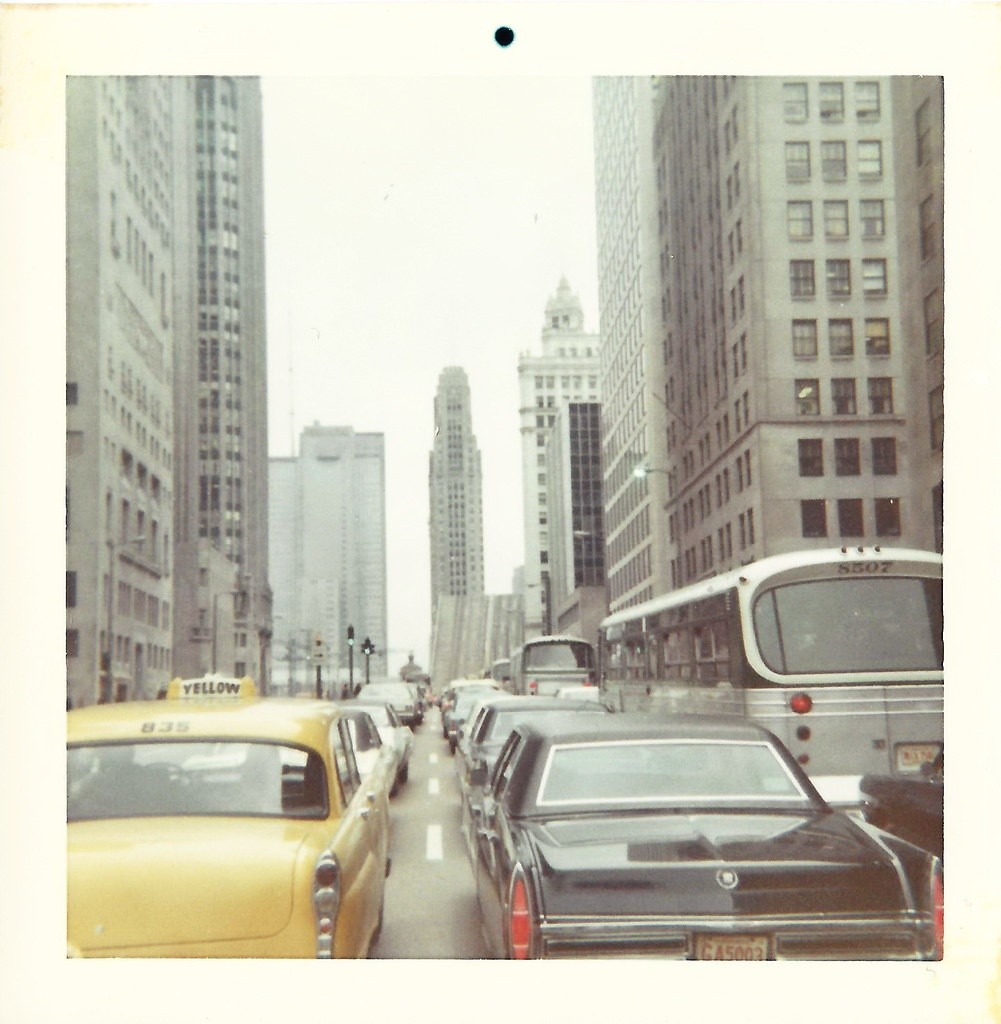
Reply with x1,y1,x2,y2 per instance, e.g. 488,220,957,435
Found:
586,545,945,807
492,657,512,688
511,636,599,699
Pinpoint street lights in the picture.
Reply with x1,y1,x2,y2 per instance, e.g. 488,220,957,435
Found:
210,588,240,676
104,535,146,703
632,467,683,588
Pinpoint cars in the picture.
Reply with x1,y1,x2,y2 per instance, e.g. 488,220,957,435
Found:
183,708,399,799
339,698,413,787
464,710,945,960
66,674,391,959
859,747,943,867
457,699,611,810
357,675,521,752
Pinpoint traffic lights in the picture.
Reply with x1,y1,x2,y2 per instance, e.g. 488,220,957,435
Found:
346,625,355,648
361,639,375,655
